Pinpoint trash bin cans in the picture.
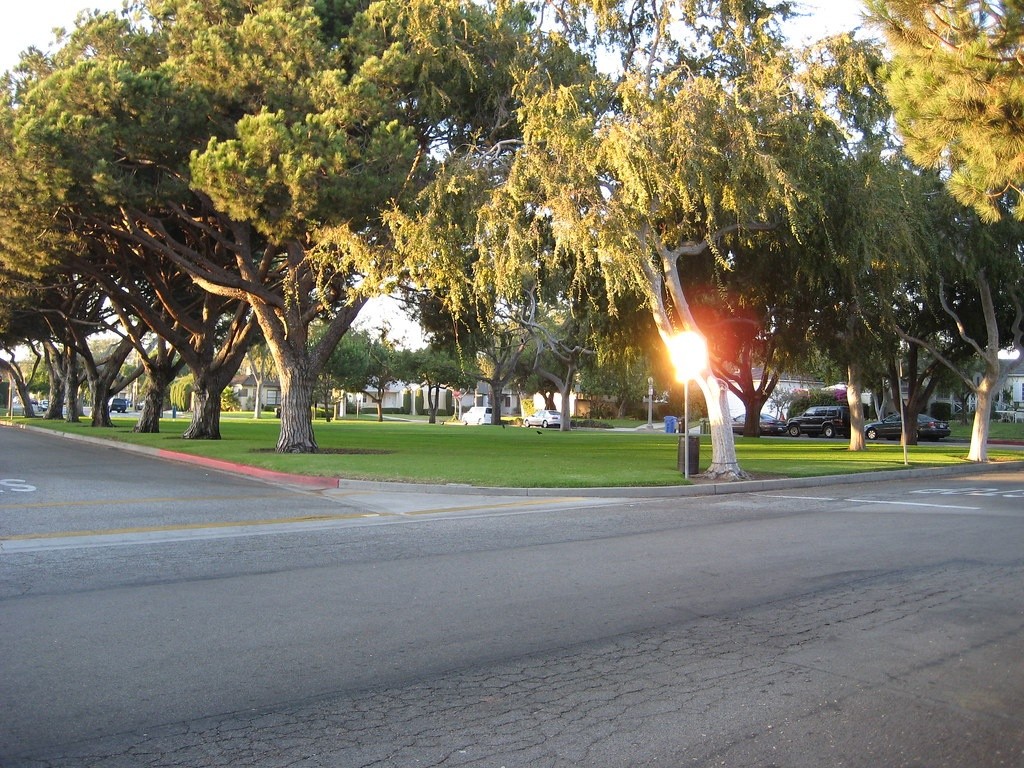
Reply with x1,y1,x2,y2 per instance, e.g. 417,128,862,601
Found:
274,407,282,419
679,416,685,433
678,435,700,476
698,418,711,435
664,414,677,433
172,405,177,418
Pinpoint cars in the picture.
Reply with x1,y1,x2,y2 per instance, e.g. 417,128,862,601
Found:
523,411,563,427
731,413,788,436
462,405,493,425
863,413,951,441
38,400,49,412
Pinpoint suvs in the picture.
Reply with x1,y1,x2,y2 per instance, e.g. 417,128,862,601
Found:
107,398,128,413
788,405,852,440
